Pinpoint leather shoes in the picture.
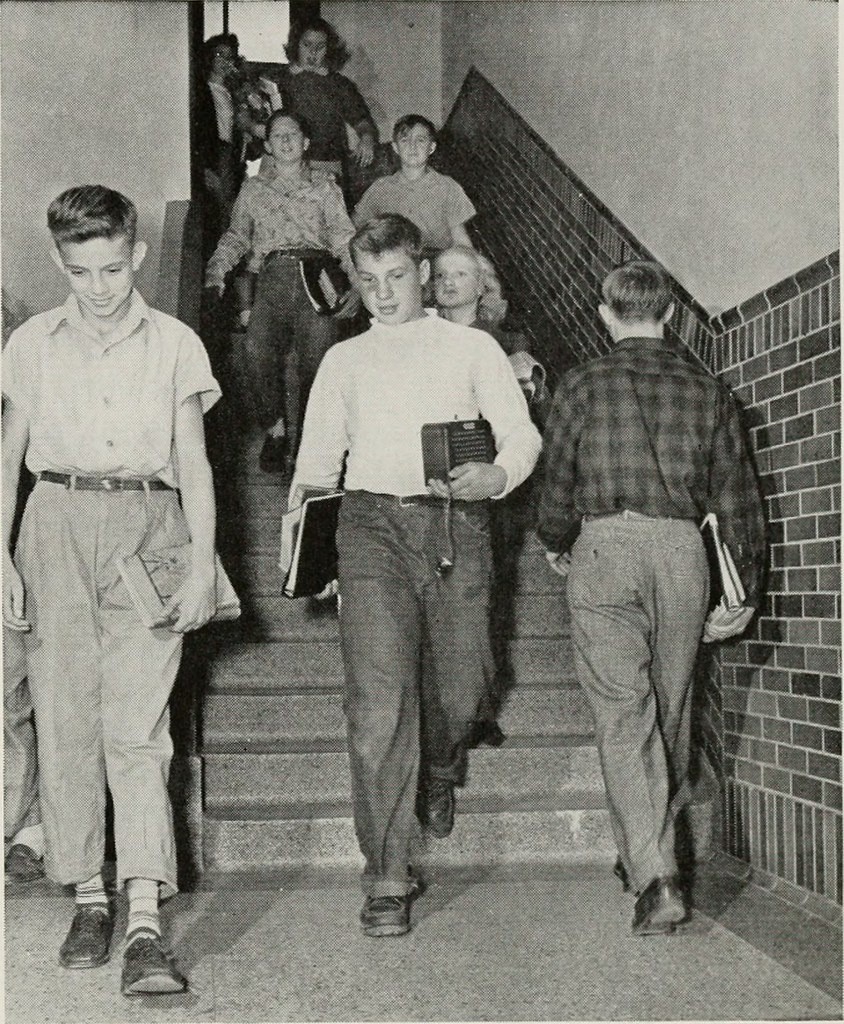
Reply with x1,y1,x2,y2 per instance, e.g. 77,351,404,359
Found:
121,936,184,995
633,876,686,936
60,899,113,969
614,859,634,892
360,895,411,936
5,844,47,884
418,787,454,838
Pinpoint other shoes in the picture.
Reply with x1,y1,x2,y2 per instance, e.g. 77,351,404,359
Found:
259,434,286,472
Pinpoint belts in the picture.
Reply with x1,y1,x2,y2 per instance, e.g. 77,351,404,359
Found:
264,249,324,266
40,470,174,491
379,493,465,508
585,510,657,521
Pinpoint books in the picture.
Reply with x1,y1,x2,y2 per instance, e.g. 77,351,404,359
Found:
701,511,747,611
296,253,349,315
276,485,340,598
112,540,241,630
246,80,284,122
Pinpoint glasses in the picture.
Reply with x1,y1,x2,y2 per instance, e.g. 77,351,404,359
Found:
215,52,235,60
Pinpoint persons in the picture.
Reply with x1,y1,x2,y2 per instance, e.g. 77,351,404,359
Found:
349,114,480,310
539,262,766,935
431,247,552,745
287,213,541,935
204,108,358,482
195,32,267,328
3,184,224,998
249,13,379,195
